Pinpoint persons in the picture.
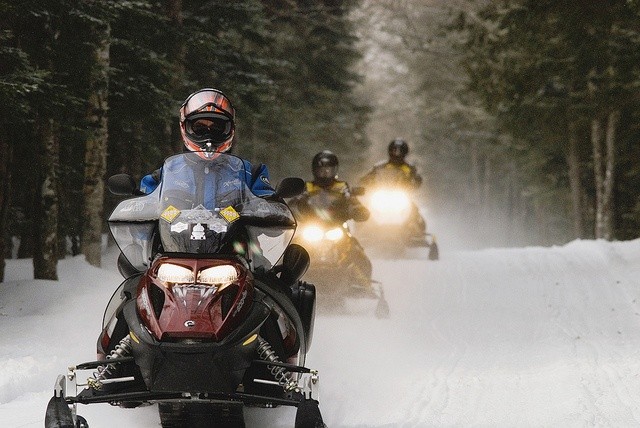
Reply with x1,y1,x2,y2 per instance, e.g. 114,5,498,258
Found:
288,149,371,287
363,139,423,188
132,87,277,280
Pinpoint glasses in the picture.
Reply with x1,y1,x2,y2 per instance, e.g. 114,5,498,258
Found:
190,122,226,136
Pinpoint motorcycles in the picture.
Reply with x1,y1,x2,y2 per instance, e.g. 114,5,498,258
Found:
45,151,325,428
347,164,438,261
289,187,390,317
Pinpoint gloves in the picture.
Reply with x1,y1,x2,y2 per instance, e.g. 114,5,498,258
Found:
254,199,295,237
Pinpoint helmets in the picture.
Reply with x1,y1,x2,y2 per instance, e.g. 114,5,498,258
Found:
311,151,339,186
388,138,409,162
178,88,238,168
